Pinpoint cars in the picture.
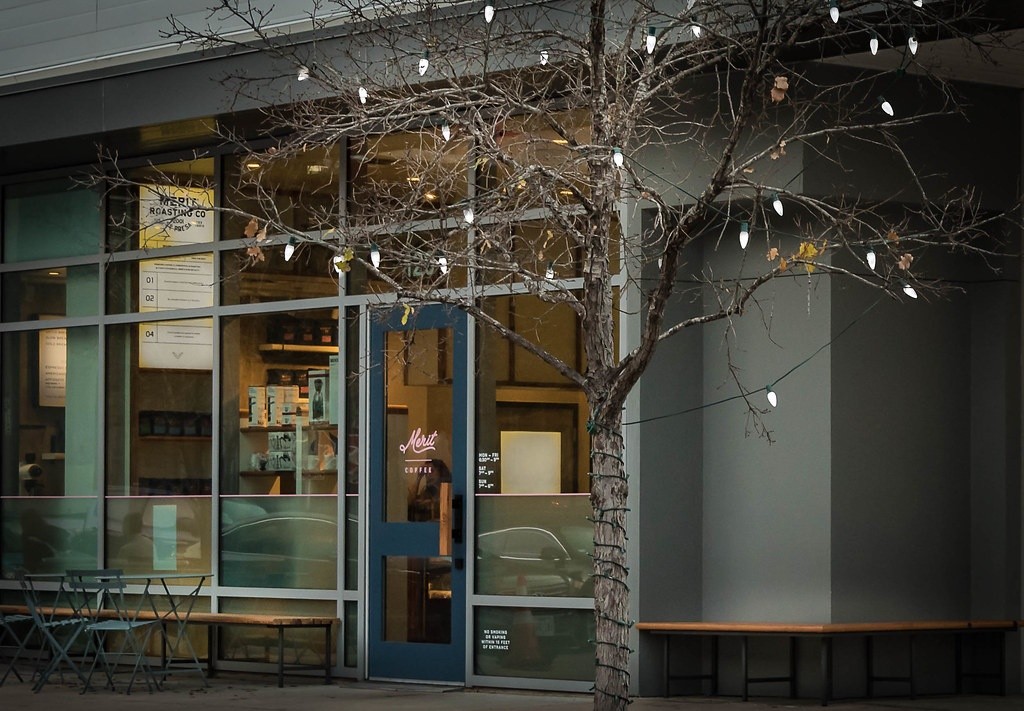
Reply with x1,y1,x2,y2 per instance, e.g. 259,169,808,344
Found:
473,512,598,653
220,511,448,650
85,486,199,557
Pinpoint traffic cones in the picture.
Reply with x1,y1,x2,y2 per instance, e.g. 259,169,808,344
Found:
498,574,540,662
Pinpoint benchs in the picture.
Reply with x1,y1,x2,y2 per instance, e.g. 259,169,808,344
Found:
0,605,341,689
635,620,1024,706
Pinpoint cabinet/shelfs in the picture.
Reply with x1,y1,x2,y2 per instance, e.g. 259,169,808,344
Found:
240,426,338,477
260,344,339,402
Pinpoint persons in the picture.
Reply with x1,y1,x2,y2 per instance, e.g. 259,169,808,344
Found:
407,459,451,509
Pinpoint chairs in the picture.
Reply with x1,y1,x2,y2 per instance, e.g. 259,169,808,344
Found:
0,569,159,695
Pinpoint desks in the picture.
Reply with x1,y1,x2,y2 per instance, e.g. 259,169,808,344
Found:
95,574,214,689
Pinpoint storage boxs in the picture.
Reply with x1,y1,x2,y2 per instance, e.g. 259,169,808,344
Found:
248,370,329,426
268,432,309,470
